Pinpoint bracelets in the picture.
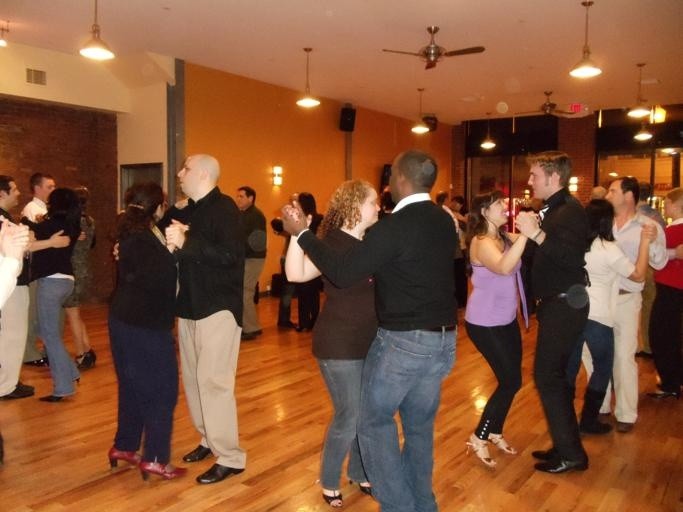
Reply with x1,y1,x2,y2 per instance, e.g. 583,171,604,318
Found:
536,230,542,238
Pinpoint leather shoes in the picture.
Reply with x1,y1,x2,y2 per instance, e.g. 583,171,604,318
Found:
139,458,188,481
1,381,35,399
241,332,256,340
278,323,297,329
646,391,680,400
196,462,245,485
598,412,612,425
108,446,143,468
182,444,211,462
26,358,48,367
534,460,588,474
252,329,262,335
39,395,63,402
616,422,633,432
532,450,552,460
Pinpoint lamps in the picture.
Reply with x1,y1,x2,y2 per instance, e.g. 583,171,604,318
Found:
480,99,498,151
294,48,321,109
77,0,113,61
624,62,667,141
410,87,433,134
1,20,10,46
272,165,283,187
567,1,602,80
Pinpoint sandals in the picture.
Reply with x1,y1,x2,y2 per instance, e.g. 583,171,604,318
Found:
349,480,371,495
322,490,343,508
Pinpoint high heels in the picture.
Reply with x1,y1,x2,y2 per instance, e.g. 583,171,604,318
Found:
74,353,92,370
465,441,497,469
85,349,96,368
487,435,518,455
296,325,308,332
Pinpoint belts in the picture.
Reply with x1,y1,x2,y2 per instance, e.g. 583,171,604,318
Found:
619,289,631,294
536,292,567,305
427,325,456,332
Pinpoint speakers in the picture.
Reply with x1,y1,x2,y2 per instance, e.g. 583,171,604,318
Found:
422,116,437,132
339,107,355,132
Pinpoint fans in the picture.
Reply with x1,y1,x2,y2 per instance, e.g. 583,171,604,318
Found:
513,89,577,118
382,25,486,70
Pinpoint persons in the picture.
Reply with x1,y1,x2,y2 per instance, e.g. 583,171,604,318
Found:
279,146,461,512
592,181,683,398
581,178,670,436
232,187,267,341
97,177,190,482
0,172,95,407
500,151,592,475
380,185,468,241
273,192,323,332
110,153,249,489
462,189,535,468
579,198,656,434
282,179,381,506
